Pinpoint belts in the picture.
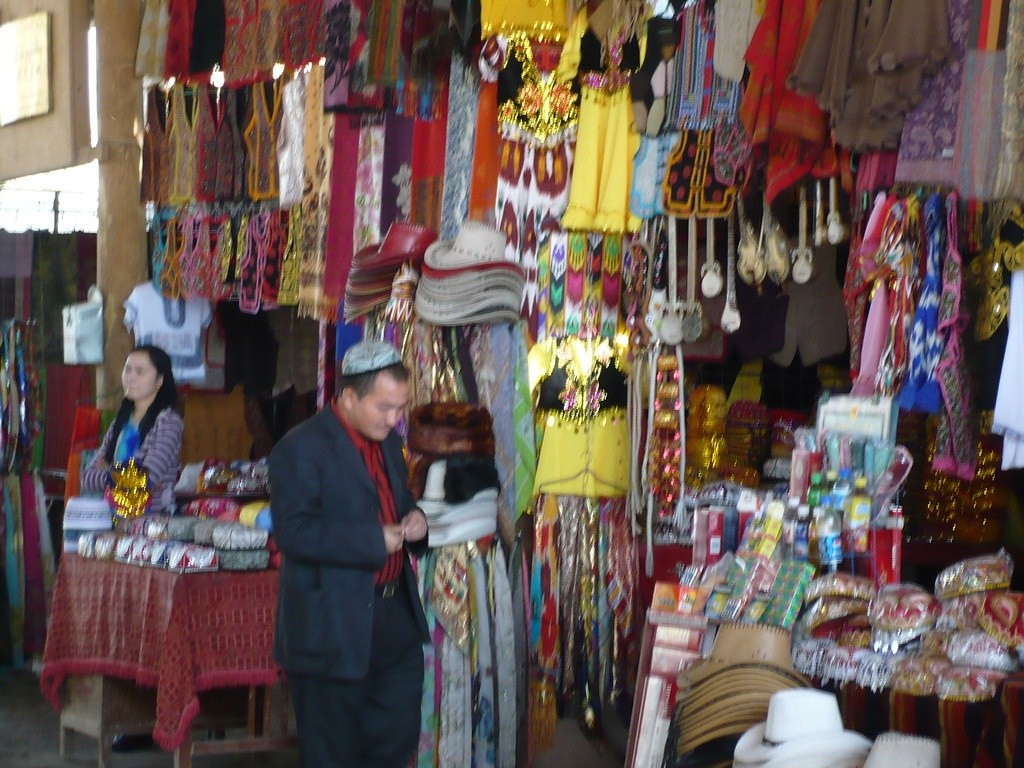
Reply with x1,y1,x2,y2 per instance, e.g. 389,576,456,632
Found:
373,581,401,601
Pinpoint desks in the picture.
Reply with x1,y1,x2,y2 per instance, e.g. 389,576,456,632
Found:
39,554,283,768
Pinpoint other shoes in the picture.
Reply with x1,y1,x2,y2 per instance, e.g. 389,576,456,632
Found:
113,733,154,751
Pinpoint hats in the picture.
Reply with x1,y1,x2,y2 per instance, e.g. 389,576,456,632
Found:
62,455,279,573
413,218,525,326
828,733,940,768
792,553,1024,700
408,404,496,456
342,341,402,376
733,689,873,768
676,623,816,758
342,221,438,326
415,456,499,547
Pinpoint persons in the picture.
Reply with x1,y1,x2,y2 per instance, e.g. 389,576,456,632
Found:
88,345,184,515
267,342,429,768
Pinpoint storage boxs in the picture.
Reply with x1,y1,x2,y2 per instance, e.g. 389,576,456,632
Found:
630,449,902,767
